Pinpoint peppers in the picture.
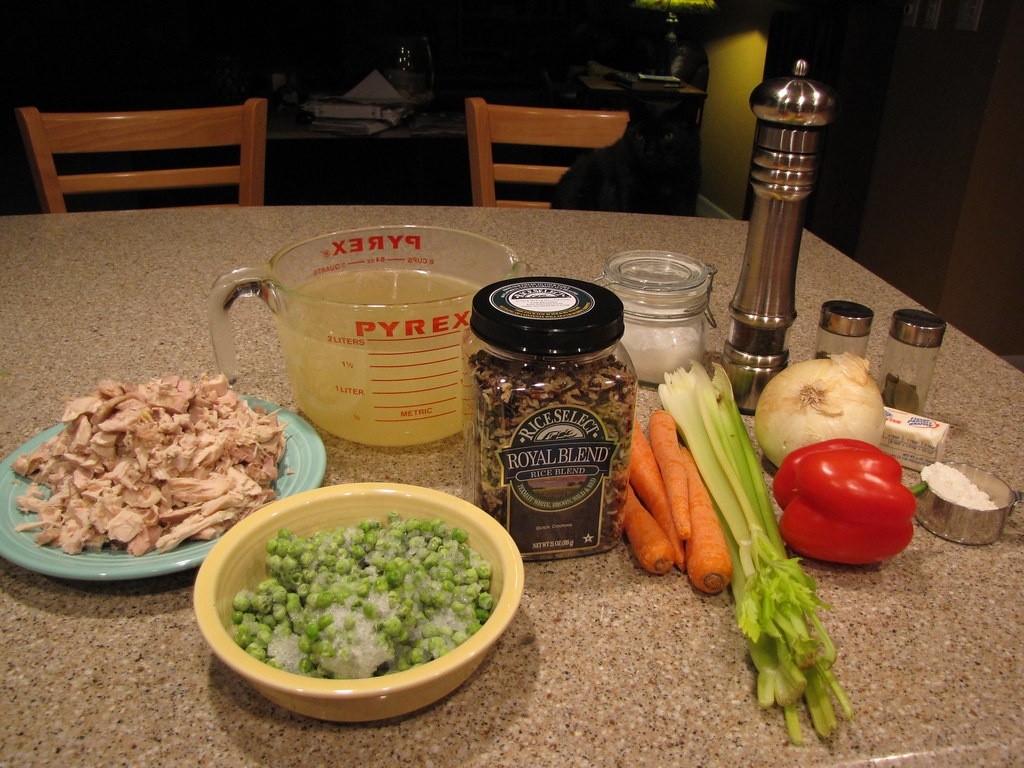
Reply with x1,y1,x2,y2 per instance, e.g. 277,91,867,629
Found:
770,437,930,566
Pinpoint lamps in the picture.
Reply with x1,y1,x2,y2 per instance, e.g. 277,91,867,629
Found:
630,0,720,77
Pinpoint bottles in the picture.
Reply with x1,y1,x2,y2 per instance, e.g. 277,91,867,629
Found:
606,250,711,387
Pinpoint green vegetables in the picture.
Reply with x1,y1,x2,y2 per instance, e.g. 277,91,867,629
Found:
656,360,855,747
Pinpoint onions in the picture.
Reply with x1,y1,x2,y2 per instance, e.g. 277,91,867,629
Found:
753,352,885,467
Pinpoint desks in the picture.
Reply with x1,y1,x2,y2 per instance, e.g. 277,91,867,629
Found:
579,77,708,159
266,109,467,139
0,204,1024,768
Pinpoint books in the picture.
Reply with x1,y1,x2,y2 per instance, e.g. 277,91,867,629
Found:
638,70,682,83
301,67,417,137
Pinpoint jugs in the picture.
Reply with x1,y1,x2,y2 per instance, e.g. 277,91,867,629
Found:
206,225,520,447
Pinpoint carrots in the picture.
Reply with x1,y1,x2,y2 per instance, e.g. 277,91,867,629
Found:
615,410,732,593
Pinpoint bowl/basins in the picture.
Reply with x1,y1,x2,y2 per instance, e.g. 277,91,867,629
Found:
194,481,525,723
914,462,1024,545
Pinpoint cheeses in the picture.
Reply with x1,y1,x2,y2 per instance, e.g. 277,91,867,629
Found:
877,403,950,472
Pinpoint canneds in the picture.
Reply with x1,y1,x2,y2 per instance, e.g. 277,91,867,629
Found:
464,275,639,561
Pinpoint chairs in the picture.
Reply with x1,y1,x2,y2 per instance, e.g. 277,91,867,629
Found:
465,97,630,213
15,99,267,214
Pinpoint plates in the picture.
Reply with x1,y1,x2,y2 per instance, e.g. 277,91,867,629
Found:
2,397,326,582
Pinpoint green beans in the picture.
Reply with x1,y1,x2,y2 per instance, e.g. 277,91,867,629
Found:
231,512,494,680
